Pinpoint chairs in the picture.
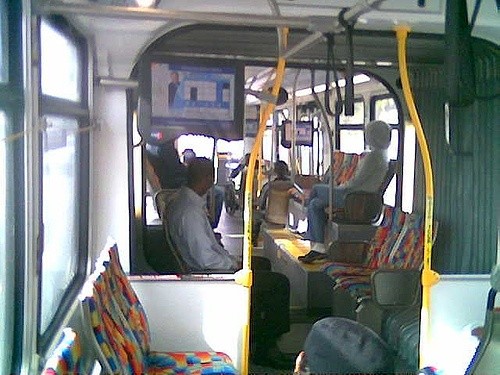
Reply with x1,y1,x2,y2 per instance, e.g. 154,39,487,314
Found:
264,190,289,226
164,219,235,274
326,160,399,258
319,205,409,288
289,151,343,229
79,268,238,375
96,238,235,365
143,152,179,219
301,152,358,221
38,327,83,375
332,213,440,304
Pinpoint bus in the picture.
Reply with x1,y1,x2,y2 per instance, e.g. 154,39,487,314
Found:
0,1,499,375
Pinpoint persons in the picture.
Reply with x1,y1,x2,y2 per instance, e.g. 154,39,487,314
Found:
298,119,393,266
163,156,297,365
292,316,395,374
231,152,250,190
257,159,293,226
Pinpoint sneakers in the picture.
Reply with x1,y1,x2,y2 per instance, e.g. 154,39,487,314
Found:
298,250,327,263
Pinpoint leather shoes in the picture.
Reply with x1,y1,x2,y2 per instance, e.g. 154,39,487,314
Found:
253,344,297,370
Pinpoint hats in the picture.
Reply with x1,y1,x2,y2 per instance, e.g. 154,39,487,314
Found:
304,317,396,375
